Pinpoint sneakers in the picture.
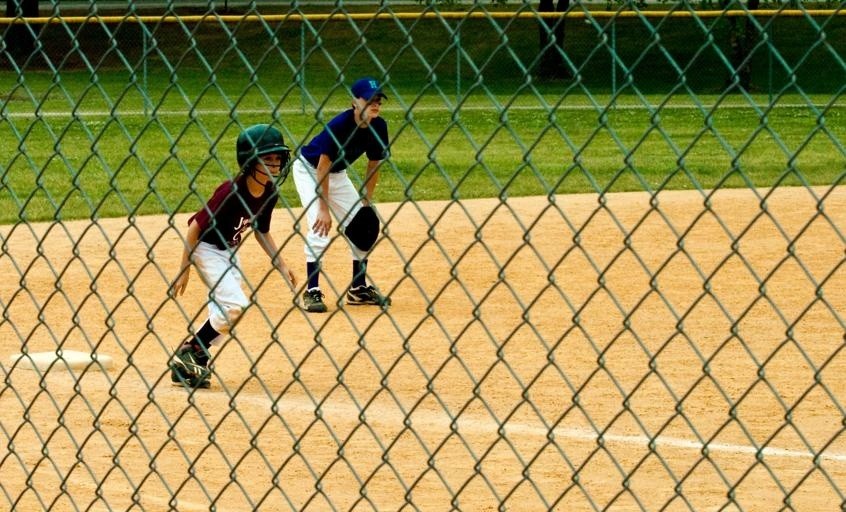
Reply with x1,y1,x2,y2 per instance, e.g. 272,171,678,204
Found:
171,365,210,388
173,342,212,379
346,285,391,305
302,290,327,312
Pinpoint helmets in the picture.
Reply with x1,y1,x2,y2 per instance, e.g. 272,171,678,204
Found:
236,123,290,187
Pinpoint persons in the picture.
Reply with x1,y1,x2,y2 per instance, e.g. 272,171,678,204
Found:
166,123,299,389
290,76,394,314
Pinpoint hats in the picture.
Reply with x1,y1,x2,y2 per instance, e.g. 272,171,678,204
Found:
352,77,387,101
